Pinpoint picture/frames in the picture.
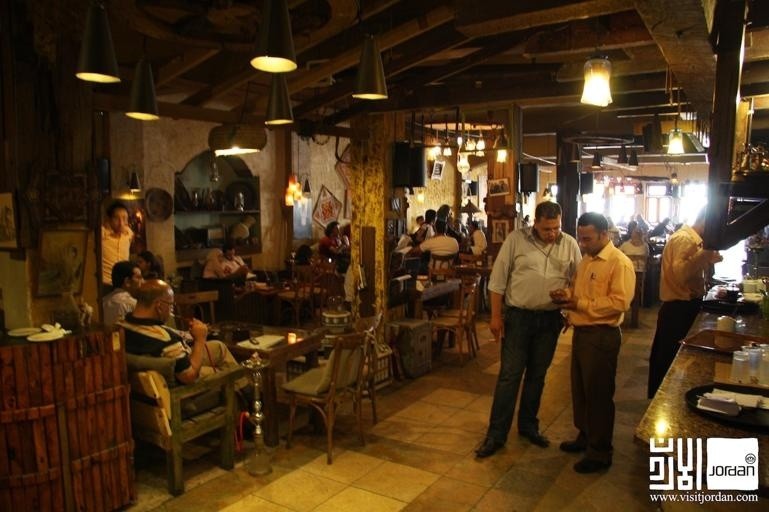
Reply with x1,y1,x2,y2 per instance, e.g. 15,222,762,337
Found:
488,178,512,196
29,228,88,297
431,160,446,181
0,188,22,252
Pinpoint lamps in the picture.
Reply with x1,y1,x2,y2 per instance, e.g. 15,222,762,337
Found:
407,109,509,175
458,198,482,225
71,1,391,195
580,52,702,200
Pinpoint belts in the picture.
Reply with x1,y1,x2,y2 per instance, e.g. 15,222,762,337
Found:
510,305,560,316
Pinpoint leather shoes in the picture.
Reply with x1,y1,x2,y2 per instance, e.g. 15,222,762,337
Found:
559,440,591,453
573,457,613,474
474,437,506,457
517,429,551,448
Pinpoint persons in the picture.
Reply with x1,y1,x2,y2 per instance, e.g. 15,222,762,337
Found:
88,201,725,475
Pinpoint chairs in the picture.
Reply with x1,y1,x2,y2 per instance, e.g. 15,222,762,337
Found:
127,222,650,498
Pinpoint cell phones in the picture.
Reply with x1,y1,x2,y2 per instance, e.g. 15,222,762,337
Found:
553,300,568,305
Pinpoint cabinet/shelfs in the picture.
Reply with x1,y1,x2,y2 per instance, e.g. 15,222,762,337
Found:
174,147,261,260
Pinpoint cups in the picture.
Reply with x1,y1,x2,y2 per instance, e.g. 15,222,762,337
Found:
728,343,769,384
716,316,735,333
713,332,736,349
726,286,739,303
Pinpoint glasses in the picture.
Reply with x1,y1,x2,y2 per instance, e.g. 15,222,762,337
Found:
160,299,176,307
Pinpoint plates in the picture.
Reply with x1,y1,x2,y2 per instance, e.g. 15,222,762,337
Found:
225,182,260,209
700,298,760,314
683,383,769,432
25,331,63,342
6,327,43,337
146,188,172,222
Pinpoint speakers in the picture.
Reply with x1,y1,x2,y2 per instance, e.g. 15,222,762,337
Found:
581,173,593,195
390,141,426,188
520,162,539,192
